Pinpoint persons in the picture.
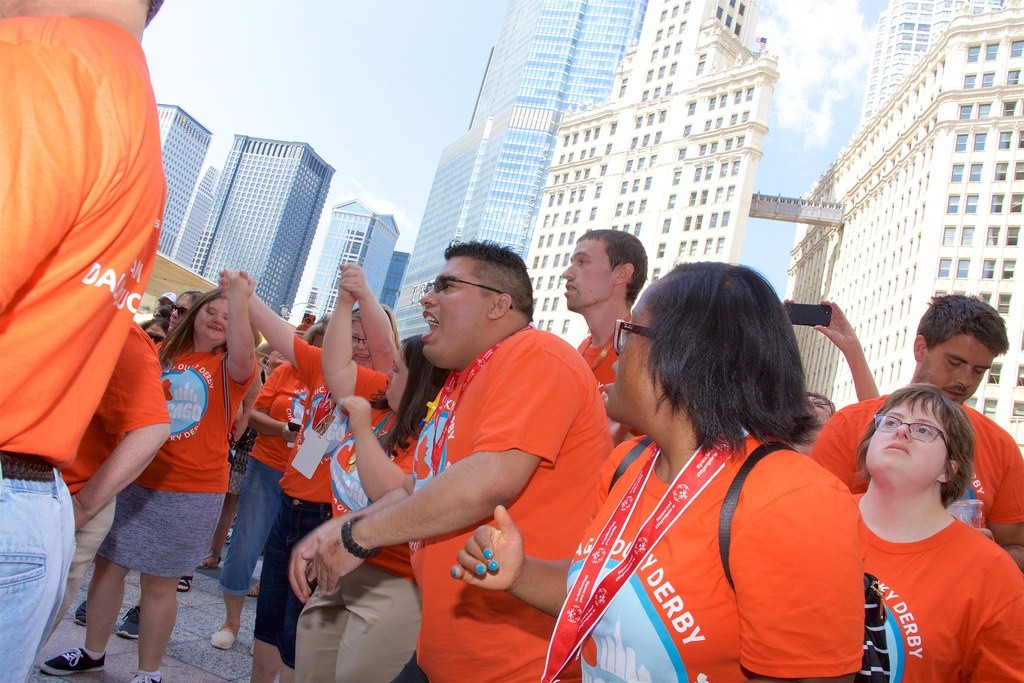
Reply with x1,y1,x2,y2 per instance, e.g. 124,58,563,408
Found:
0,0,169,683
38,229,1024,683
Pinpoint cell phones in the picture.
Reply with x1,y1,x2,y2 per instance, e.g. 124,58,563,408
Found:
785,303,833,326
288,422,302,432
299,313,316,331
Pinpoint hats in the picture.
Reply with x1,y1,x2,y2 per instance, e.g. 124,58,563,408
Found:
158,292,177,304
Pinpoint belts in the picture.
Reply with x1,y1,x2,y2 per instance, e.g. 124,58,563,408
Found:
1,449,58,482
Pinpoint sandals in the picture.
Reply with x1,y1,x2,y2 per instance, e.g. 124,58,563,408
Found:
196,553,222,568
176,575,193,591
247,582,260,596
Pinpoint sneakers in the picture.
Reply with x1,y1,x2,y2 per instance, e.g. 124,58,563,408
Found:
74,599,87,626
117,606,140,639
133,671,163,683
210,625,237,649
40,647,106,676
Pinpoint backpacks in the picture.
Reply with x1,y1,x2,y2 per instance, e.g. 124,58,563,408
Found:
719,442,892,683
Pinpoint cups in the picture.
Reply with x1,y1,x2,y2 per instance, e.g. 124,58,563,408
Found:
949,498,984,529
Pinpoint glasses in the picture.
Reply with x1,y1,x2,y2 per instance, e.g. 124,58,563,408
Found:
813,397,833,418
424,276,514,312
612,318,658,356
148,332,162,344
352,336,368,349
171,305,187,317
874,413,948,456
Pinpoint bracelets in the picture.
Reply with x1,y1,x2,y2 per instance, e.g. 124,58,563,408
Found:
339,515,380,560
